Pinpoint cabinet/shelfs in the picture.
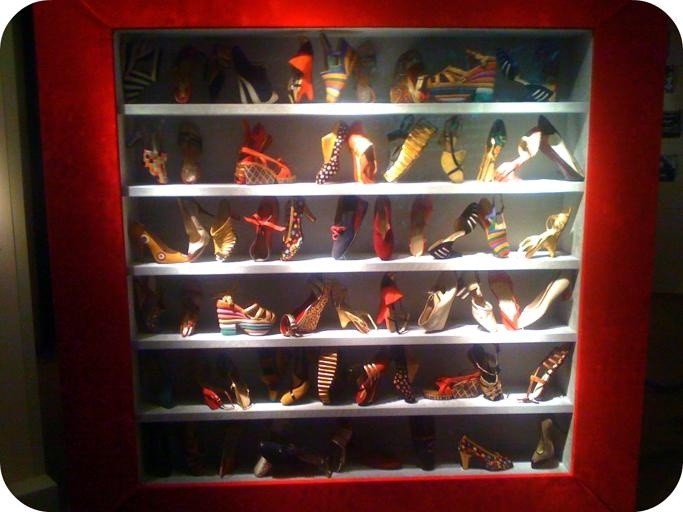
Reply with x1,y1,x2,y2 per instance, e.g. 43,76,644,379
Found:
109,26,596,483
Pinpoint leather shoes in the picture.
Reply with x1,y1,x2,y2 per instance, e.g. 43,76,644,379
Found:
517,270,577,329
488,270,522,330
476,119,506,181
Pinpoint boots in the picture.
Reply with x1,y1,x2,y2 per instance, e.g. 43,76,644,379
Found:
143,366,173,408
206,354,250,410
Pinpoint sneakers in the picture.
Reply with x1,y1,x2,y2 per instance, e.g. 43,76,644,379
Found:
538,115,585,181
347,120,379,182
417,271,458,333
121,39,160,103
407,197,432,257
260,440,324,473
373,195,394,261
315,119,349,183
330,195,369,259
243,196,287,261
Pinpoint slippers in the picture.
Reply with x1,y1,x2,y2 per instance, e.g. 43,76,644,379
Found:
176,278,202,337
177,122,203,183
328,420,352,473
134,275,160,333
383,114,438,182
286,40,315,103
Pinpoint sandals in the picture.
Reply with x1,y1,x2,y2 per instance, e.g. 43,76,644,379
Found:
320,33,359,103
516,207,571,259
375,271,410,334
389,48,431,102
526,343,569,402
477,195,511,258
423,368,481,400
331,280,377,333
467,344,503,401
279,275,331,337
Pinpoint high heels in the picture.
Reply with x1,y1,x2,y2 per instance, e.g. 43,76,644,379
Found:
128,218,189,263
230,46,278,104
427,201,481,259
355,38,377,102
233,117,297,184
437,113,465,183
430,49,497,101
360,442,400,469
206,44,225,100
209,199,240,263
531,419,562,465
354,345,392,406
457,434,513,472
387,345,415,403
280,348,309,405
497,131,542,177
176,196,216,263
496,47,558,101
280,196,316,261
457,271,499,331
128,117,169,183
216,295,278,336
